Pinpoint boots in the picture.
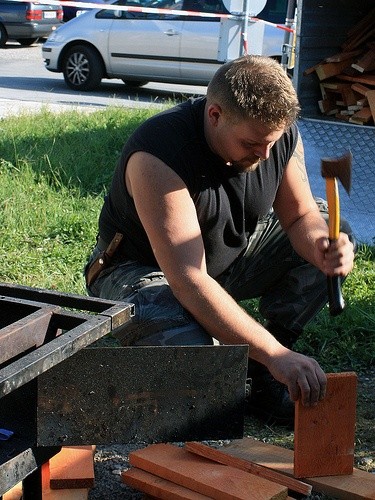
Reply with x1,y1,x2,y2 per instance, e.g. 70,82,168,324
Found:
244,326,302,428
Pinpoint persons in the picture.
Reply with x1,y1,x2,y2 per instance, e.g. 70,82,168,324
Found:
83,54,357,430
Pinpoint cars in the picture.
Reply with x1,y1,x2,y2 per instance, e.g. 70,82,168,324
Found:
0,0,65,48
41,0,297,93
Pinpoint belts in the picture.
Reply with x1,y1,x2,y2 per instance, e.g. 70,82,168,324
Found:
97,238,126,262
